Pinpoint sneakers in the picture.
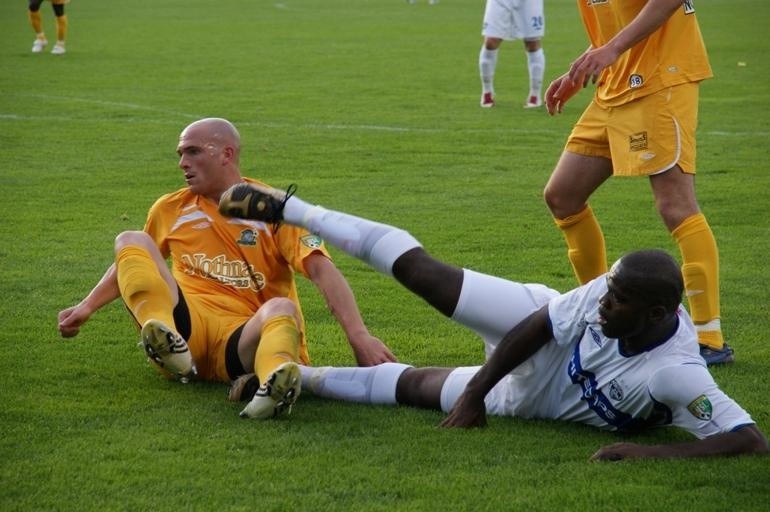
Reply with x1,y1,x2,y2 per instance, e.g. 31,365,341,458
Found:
228,361,302,421
137,319,199,384
698,343,737,367
480,91,495,110
51,45,67,55
526,94,541,107
217,184,298,234
32,38,48,53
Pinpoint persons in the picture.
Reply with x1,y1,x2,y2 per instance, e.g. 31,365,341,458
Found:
58,117,399,420
542,1,735,366
29,1,68,55
479,1,545,109
217,180,769,463
